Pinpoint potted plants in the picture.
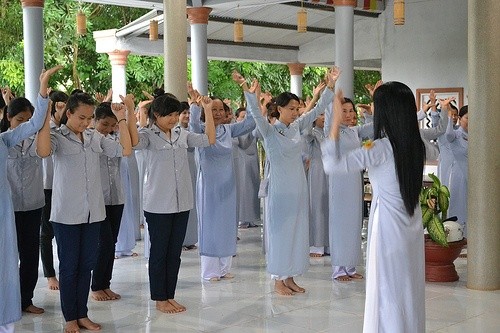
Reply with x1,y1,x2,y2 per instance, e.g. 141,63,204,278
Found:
414,173,464,282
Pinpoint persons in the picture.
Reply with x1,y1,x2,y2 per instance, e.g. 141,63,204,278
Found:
0,65,469,333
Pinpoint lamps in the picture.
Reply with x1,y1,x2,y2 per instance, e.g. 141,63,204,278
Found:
77,0,87,34
234,0,243,42
298,0,307,33
394,0,405,25
150,5,158,41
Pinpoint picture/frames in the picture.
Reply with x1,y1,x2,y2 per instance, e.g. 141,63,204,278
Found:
415,87,464,128
